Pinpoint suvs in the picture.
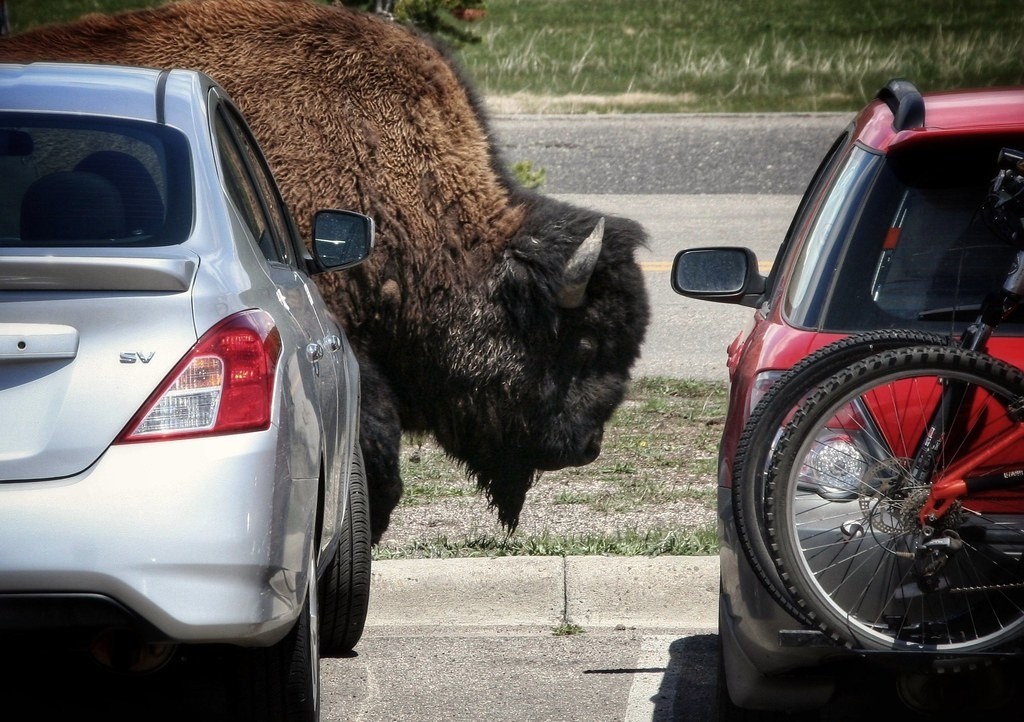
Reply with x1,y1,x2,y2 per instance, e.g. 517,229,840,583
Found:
0,62,377,722
671,79,1024,722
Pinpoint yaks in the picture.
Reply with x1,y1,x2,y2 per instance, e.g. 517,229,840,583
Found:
0,0,652,550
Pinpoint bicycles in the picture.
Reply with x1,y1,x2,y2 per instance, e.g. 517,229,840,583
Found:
730,145,1024,666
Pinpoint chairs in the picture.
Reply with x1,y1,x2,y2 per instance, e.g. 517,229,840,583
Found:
19,170,126,243
